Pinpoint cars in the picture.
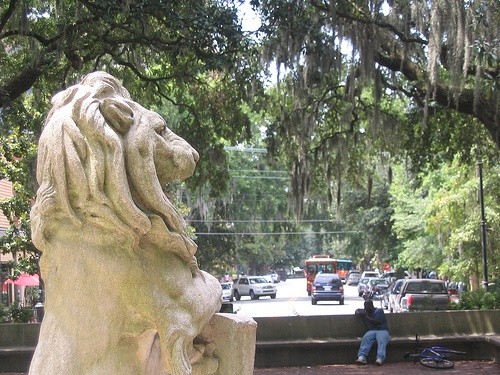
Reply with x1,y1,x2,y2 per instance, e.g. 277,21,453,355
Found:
347,274,361,286
271,274,280,283
263,275,274,283
221,283,233,302
364,279,451,311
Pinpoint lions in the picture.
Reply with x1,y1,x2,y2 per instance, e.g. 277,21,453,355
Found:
30,71,222,375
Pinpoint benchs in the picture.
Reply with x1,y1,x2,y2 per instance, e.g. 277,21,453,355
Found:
1,323,43,372
252,309,500,368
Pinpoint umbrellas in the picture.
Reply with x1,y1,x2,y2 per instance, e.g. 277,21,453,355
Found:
3,273,40,308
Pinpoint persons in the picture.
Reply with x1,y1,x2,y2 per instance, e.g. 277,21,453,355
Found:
355,300,391,366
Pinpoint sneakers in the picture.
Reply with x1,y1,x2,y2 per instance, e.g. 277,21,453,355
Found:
376,357,382,366
356,356,367,365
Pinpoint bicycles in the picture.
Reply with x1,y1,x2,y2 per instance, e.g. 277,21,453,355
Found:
404,334,466,368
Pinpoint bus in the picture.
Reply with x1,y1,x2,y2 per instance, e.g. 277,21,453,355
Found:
307,255,352,296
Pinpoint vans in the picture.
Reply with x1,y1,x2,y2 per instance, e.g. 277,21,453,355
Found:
358,272,381,295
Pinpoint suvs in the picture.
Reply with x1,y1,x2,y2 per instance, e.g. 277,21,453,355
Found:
232,277,276,300
311,273,344,305
